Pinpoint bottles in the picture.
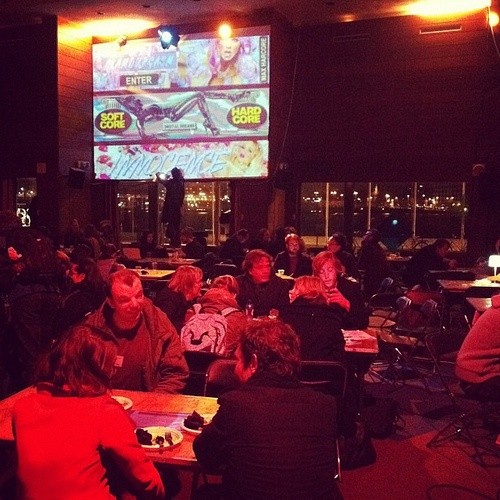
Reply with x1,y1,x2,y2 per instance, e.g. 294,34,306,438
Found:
246,299,254,318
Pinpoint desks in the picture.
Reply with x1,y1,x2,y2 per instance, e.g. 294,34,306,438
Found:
342,329,381,446
139,256,201,265
126,267,175,290
465,297,492,325
387,254,409,281
436,277,475,348
0,381,221,500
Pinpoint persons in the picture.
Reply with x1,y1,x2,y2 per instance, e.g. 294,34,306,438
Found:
402,238,457,287
191,37,251,86
193,316,343,500
175,274,247,358
212,140,267,177
0,324,166,500
280,276,345,363
156,168,185,247
153,264,203,323
312,250,370,331
455,294,500,446
71,270,191,394
234,250,291,318
357,228,388,295
273,233,314,278
0,217,500,500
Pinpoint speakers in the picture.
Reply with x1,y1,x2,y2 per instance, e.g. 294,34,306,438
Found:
68,167,85,189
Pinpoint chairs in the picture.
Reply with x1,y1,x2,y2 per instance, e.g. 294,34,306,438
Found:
0,238,500,500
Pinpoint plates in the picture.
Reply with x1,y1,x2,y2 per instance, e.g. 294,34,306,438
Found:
112,395,133,410
135,426,183,448
182,413,216,434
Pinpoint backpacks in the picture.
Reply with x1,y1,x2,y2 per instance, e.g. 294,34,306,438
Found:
181,304,238,355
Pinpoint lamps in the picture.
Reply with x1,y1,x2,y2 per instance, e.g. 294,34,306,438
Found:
158,26,183,49
487,254,500,277
216,19,235,39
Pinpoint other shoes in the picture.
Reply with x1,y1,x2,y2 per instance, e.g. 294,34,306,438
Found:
481,420,500,435
